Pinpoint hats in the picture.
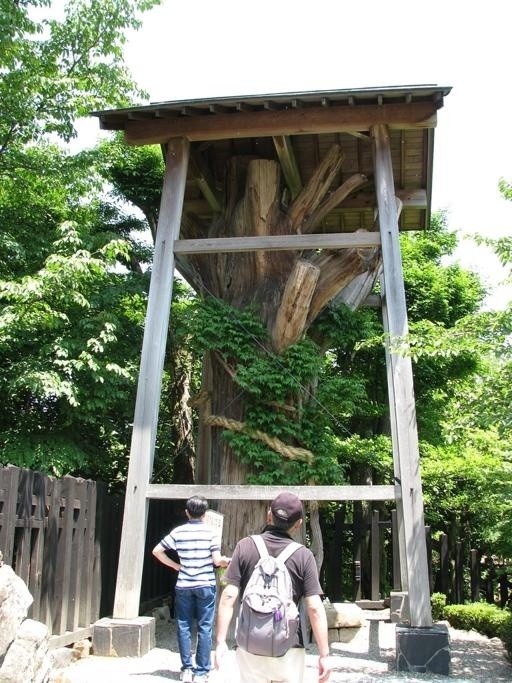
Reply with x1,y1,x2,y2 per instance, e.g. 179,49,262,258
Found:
270,491,304,521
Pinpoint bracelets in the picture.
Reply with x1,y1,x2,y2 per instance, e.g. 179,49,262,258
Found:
320,654,329,657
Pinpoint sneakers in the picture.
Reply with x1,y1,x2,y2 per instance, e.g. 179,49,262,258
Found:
179,669,192,683
194,675,208,683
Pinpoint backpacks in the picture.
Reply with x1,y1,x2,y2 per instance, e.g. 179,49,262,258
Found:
234,535,306,658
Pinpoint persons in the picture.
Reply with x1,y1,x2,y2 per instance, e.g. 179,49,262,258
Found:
215,492,332,683
152,495,229,683
